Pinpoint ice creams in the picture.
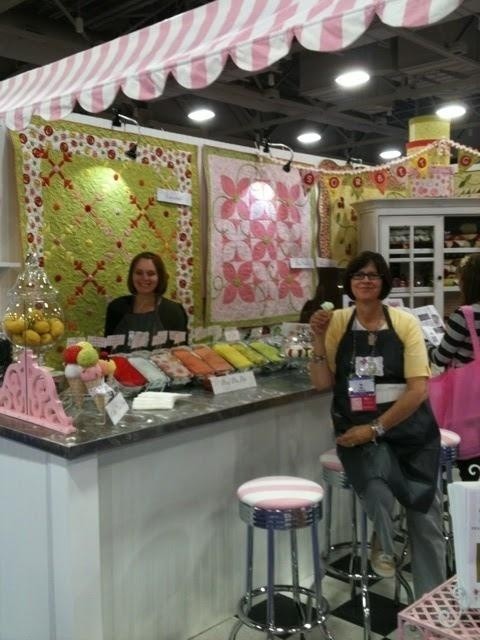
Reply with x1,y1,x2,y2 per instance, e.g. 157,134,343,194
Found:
63,341,117,413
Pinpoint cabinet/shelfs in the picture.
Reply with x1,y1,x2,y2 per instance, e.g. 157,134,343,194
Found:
350,197,480,326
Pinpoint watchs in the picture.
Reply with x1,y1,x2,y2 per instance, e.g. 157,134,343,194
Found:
372,418,386,440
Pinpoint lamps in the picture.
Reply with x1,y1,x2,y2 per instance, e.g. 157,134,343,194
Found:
262,136,294,173
109,107,141,161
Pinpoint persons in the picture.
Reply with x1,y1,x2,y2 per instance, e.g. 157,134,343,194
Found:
300,264,351,326
308,251,449,594
101,252,188,344
422,252,480,486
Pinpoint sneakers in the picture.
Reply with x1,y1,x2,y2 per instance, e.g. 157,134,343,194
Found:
370,532,395,578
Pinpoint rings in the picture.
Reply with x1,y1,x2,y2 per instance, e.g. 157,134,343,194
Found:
351,442,354,448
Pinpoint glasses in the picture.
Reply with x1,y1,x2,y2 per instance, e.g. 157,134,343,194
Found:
352,272,383,280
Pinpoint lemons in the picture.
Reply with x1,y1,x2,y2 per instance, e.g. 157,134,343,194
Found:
3,310,65,346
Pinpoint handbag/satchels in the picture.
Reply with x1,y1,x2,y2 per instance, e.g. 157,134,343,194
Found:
428,359,480,460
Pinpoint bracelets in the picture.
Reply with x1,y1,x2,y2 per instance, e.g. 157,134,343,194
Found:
311,354,328,361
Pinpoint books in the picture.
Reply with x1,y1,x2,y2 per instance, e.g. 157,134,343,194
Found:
398,298,451,348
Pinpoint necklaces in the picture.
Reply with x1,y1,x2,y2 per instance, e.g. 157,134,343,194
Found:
359,313,385,349
